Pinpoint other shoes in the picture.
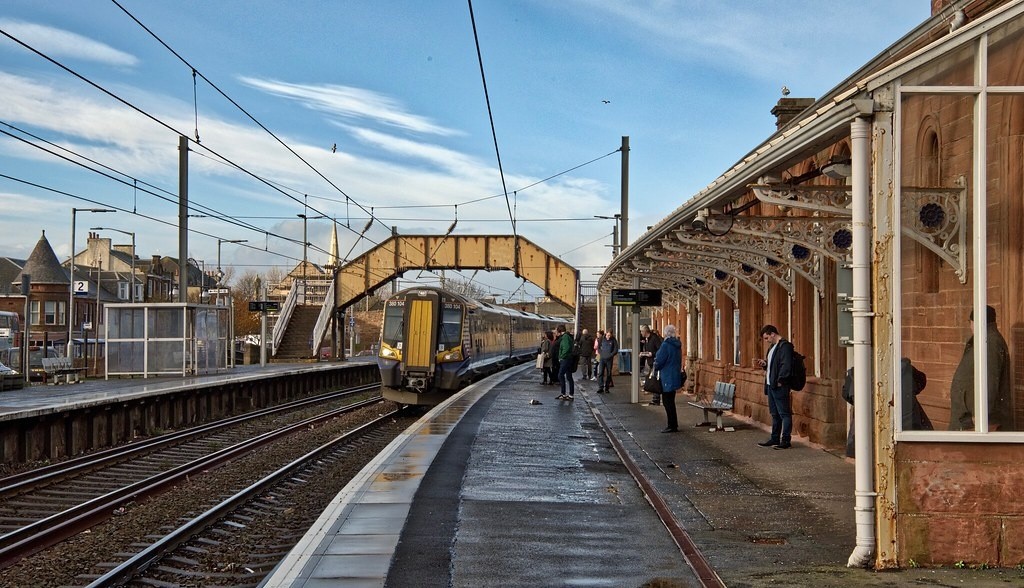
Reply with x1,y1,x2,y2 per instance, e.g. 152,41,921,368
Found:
591,376,597,382
540,381,561,385
649,402,660,406
757,440,780,447
597,388,602,393
564,396,574,401
773,442,791,450
661,427,677,433
555,395,565,400
605,390,609,393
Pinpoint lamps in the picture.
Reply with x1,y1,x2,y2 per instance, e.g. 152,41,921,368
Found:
824,164,852,181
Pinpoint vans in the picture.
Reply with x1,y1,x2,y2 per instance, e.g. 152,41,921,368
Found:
0,347,63,379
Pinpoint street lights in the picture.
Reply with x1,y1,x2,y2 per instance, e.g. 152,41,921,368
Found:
217,239,249,368
297,213,306,306
68,209,117,381
595,214,621,258
198,260,204,292
90,227,135,304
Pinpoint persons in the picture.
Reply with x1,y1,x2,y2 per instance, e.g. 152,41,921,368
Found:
843,348,934,459
639,324,664,406
653,325,682,432
540,325,618,399
756,325,792,450
949,305,1010,431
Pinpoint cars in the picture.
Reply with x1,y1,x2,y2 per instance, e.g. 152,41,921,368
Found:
0,361,24,392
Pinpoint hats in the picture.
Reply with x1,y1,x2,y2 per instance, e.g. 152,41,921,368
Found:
970,305,996,323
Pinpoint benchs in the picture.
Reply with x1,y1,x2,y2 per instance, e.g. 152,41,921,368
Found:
688,380,736,430
41,356,90,384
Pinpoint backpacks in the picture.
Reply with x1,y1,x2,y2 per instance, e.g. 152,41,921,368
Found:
779,341,807,391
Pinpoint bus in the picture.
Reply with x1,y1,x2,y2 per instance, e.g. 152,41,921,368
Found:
0,311,22,368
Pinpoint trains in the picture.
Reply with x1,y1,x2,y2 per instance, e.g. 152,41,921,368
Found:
375,286,574,412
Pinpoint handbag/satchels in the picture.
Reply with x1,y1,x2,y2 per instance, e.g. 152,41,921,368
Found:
536,354,545,369
644,366,663,394
680,371,688,387
